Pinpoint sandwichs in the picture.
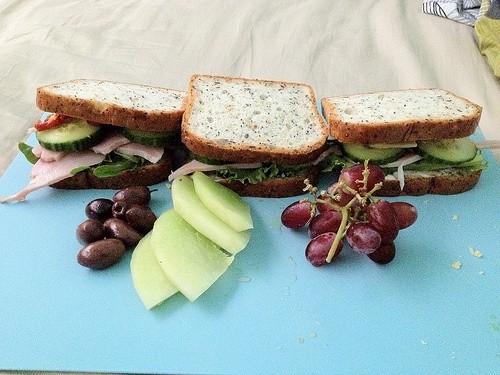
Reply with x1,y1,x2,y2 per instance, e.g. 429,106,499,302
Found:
33,76,186,190
322,86,485,198
182,74,330,198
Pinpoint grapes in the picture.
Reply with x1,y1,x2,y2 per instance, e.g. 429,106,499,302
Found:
281,162,418,265
